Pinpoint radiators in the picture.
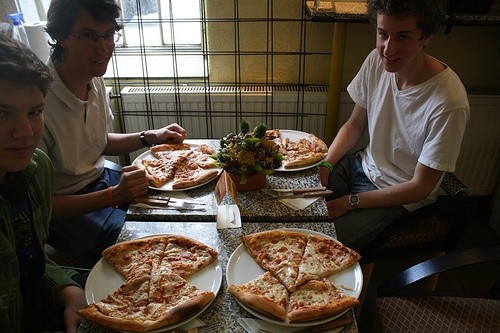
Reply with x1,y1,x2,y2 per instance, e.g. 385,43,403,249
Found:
102,88,119,166
117,86,500,195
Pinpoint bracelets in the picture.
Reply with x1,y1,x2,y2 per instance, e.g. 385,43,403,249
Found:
319,162,333,172
108,186,116,209
139,130,150,147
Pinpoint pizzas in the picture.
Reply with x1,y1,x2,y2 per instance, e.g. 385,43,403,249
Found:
136,143,219,188
227,230,361,322
76,235,219,333
266,129,328,168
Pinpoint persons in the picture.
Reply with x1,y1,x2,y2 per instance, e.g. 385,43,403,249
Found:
0,38,86,333
319,0,473,264
37,0,188,268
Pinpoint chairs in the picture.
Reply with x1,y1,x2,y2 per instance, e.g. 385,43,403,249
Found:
363,170,500,333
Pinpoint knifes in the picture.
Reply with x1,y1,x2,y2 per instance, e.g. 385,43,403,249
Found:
134,195,206,210
267,189,333,200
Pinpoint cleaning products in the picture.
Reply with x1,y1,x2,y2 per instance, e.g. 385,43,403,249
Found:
7,12,29,47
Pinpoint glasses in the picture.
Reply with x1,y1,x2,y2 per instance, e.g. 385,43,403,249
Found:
66,30,121,46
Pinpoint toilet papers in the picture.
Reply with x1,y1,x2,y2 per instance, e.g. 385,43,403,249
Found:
23,20,53,65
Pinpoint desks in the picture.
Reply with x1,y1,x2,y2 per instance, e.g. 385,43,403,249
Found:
79,219,359,333
127,135,332,217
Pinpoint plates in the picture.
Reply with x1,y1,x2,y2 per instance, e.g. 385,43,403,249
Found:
265,129,329,171
85,233,222,332
131,143,223,191
227,228,363,326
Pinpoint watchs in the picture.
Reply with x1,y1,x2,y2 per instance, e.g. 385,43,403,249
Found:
349,194,360,209
